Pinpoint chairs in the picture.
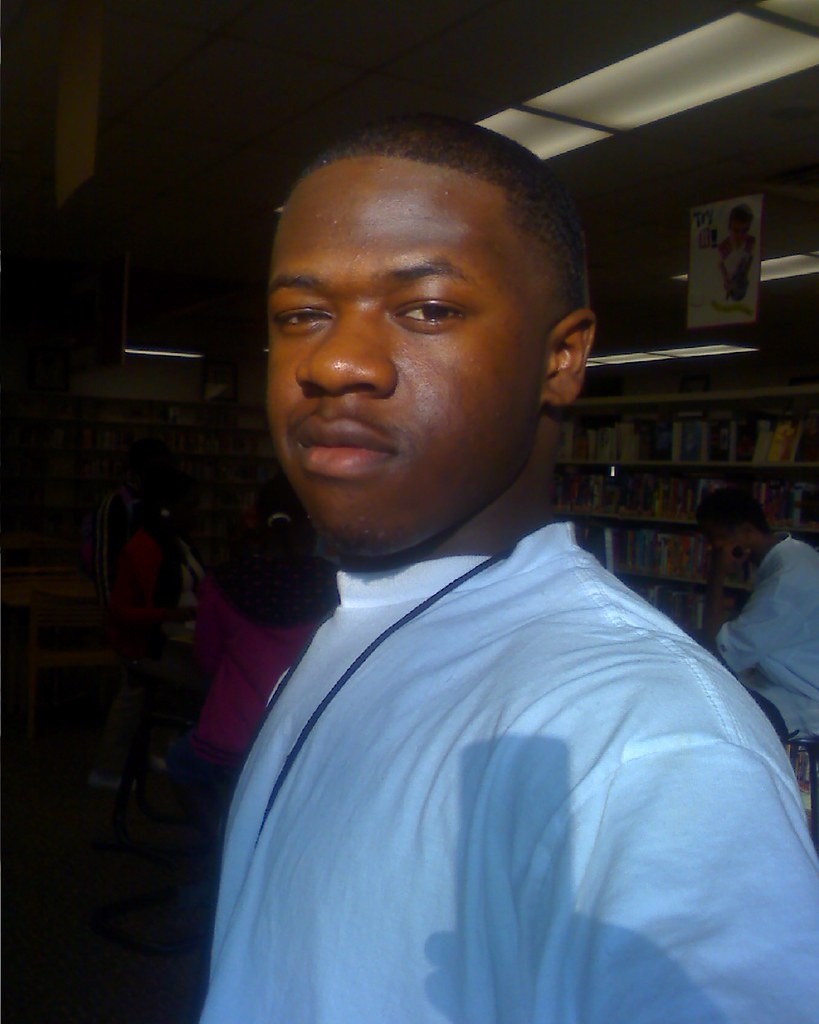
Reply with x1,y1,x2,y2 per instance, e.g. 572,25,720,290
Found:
25,587,115,746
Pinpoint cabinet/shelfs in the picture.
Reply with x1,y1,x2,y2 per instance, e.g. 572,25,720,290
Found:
552,383,819,592
0,387,268,562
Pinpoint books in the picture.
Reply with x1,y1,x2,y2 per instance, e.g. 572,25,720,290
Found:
1,409,819,638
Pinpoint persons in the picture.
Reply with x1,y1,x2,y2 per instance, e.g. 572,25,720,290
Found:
107,475,205,621
200,121,818,1024
696,486,819,745
717,204,756,301
93,465,143,596
188,479,340,768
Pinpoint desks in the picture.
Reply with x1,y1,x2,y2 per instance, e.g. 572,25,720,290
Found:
2,574,98,715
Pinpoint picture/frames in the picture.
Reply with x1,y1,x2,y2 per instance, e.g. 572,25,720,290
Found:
200,358,241,402
